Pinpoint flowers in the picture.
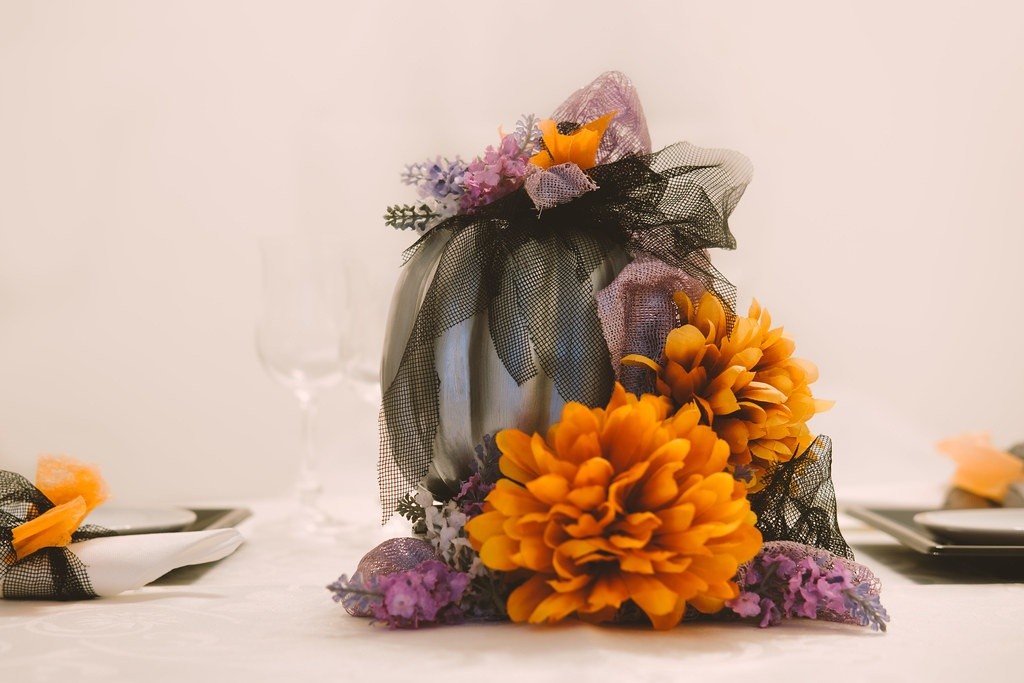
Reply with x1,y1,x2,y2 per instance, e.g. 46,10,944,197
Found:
934,430,1024,508
326,68,897,636
0,449,256,604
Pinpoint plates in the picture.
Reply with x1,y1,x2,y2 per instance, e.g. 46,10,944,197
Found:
913,507,1024,543
845,500,1024,558
